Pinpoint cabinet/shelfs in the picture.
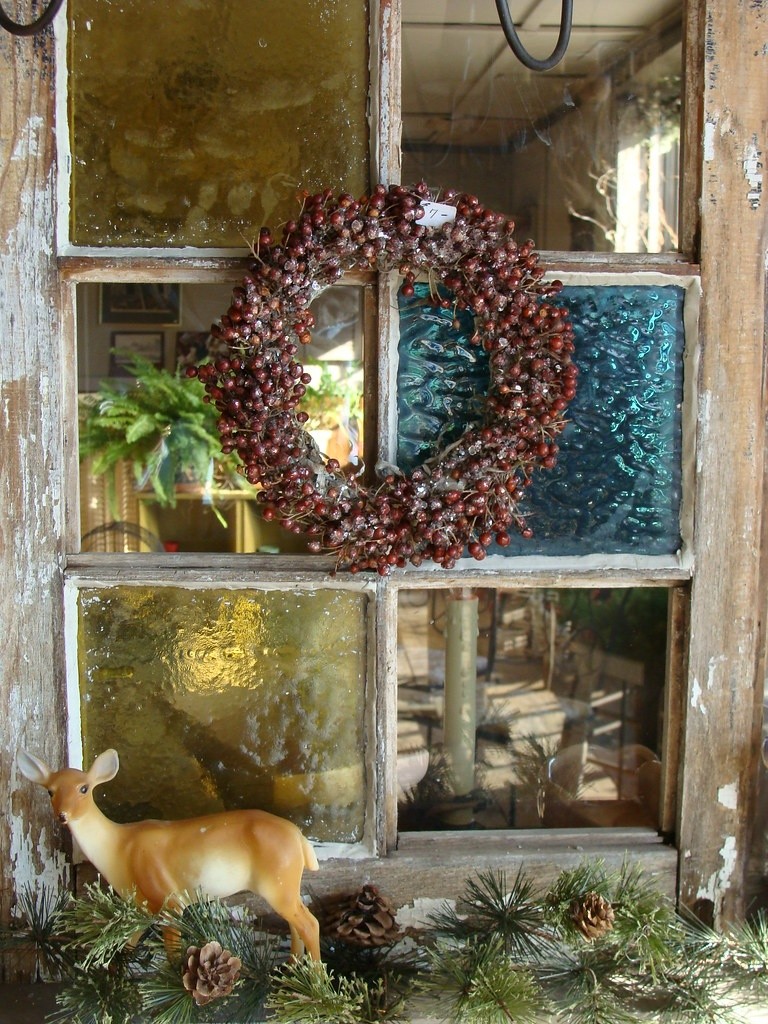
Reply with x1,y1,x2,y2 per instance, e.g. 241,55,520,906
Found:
136,484,322,554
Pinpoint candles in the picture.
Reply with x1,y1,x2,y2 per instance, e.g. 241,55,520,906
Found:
445,587,478,797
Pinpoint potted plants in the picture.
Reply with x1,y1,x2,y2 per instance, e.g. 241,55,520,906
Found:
295,354,347,462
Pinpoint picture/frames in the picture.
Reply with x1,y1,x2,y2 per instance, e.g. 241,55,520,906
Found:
110,331,165,368
99,282,183,328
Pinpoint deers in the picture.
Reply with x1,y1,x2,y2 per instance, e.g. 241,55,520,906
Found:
16,747,321,972
